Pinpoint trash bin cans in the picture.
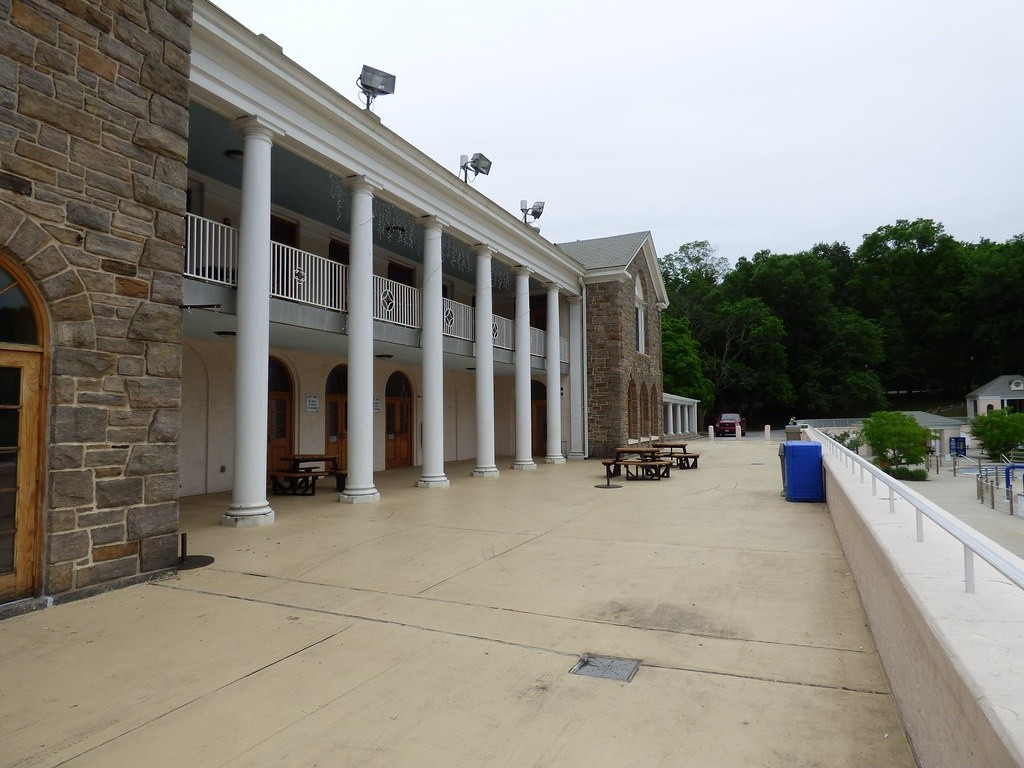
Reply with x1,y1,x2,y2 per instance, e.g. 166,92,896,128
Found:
785,425,801,441
777,440,824,503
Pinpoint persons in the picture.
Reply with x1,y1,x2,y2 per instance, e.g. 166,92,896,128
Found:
789,417,796,425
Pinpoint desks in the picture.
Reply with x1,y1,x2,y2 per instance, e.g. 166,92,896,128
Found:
280,454,340,491
651,443,691,469
612,448,660,478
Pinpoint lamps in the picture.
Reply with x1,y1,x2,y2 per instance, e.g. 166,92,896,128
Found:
459,153,492,183
224,150,243,161
356,64,396,110
520,200,545,224
214,331,236,339
375,355,394,360
386,226,405,233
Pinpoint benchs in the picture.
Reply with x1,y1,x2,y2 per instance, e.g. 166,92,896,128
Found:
270,466,347,497
601,452,700,481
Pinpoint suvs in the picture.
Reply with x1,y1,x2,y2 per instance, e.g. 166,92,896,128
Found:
715,413,747,437
